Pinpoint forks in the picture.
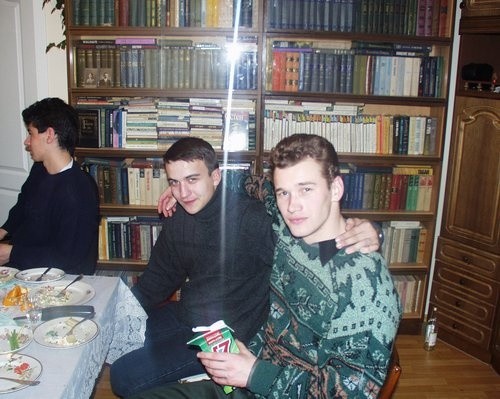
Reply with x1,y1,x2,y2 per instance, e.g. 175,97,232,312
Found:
63,312,96,337
55,274,84,298
35,267,52,281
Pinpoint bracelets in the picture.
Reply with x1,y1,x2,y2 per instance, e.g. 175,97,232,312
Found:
371,222,385,245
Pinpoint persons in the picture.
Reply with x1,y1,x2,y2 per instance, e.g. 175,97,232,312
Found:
0,96,100,277
108,136,385,399
123,133,404,399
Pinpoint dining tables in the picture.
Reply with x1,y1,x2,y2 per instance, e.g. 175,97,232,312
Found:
0,267,122,399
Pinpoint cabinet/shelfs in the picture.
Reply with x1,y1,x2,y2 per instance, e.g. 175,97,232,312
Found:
431,0,500,370
64,1,459,335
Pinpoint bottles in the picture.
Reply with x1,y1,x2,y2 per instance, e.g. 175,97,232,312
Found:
423,306,439,351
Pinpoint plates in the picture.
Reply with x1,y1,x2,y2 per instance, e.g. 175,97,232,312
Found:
28,280,95,308
0,326,34,355
0,267,21,284
0,353,43,394
13,267,65,283
33,316,99,349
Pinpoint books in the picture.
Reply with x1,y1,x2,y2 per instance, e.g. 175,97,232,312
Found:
261,163,274,176
263,99,439,157
73,36,258,91
218,160,254,172
170,287,183,301
381,220,425,264
265,0,455,40
81,156,170,208
76,97,256,152
97,270,144,288
268,37,444,98
99,218,162,265
338,161,432,212
392,272,429,319
72,0,251,27
